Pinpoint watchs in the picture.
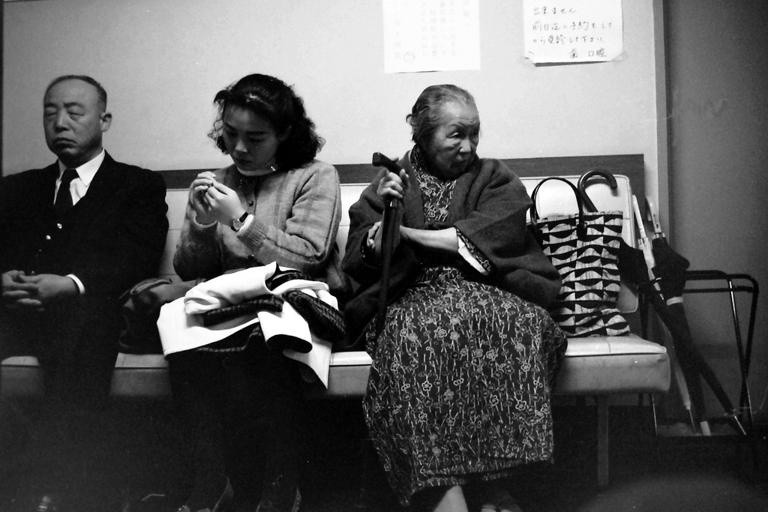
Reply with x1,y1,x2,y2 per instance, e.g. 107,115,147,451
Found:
230,211,249,233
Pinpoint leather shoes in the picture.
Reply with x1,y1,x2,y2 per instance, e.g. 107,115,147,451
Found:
34,474,304,512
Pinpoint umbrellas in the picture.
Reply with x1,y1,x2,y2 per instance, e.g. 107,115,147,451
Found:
577,166,748,438
631,190,698,434
643,193,716,440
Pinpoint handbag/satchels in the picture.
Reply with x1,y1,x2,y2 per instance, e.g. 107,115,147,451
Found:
521,178,633,339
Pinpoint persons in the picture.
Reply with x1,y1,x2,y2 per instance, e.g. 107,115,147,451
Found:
0,75,169,512
339,83,569,512
157,73,346,511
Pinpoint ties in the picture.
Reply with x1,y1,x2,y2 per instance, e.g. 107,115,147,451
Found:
52,168,80,214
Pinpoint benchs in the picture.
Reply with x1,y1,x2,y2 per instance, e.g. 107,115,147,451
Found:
2,155,671,487
